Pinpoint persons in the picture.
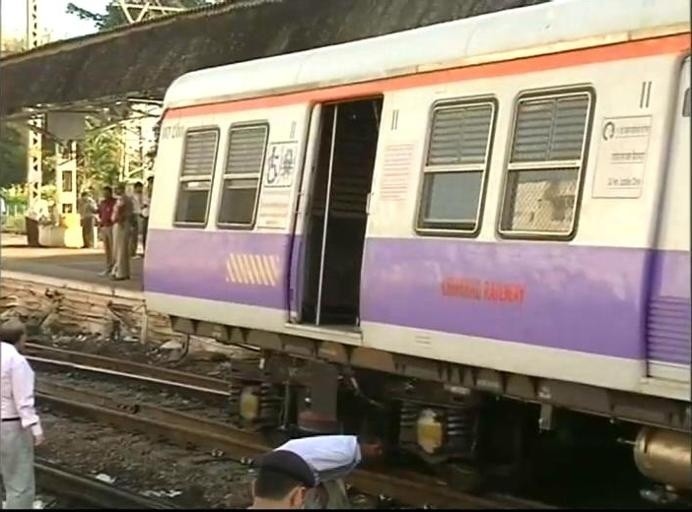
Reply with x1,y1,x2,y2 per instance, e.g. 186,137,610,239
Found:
239,417,402,512
1,314,46,511
74,176,154,281
24,197,55,247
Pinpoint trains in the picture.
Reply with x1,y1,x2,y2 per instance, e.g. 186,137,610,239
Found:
142,0,692,494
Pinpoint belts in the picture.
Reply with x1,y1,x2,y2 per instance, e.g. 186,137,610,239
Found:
1,417,22,423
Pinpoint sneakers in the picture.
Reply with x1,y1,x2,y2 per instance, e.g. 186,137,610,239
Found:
97,267,129,281
